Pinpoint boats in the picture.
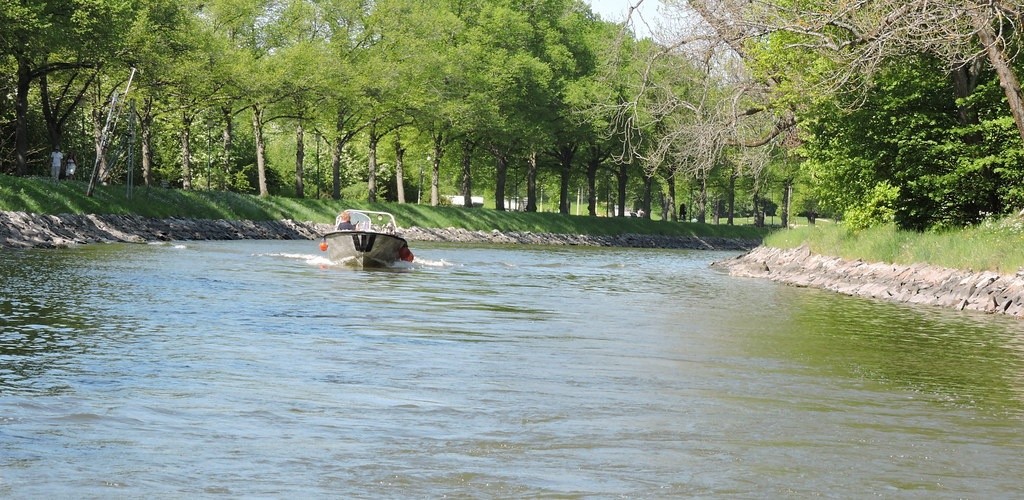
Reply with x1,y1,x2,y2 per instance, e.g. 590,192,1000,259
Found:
318,209,414,271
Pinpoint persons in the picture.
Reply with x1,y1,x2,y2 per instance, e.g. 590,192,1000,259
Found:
50,147,63,181
65,154,76,177
337,212,358,230
